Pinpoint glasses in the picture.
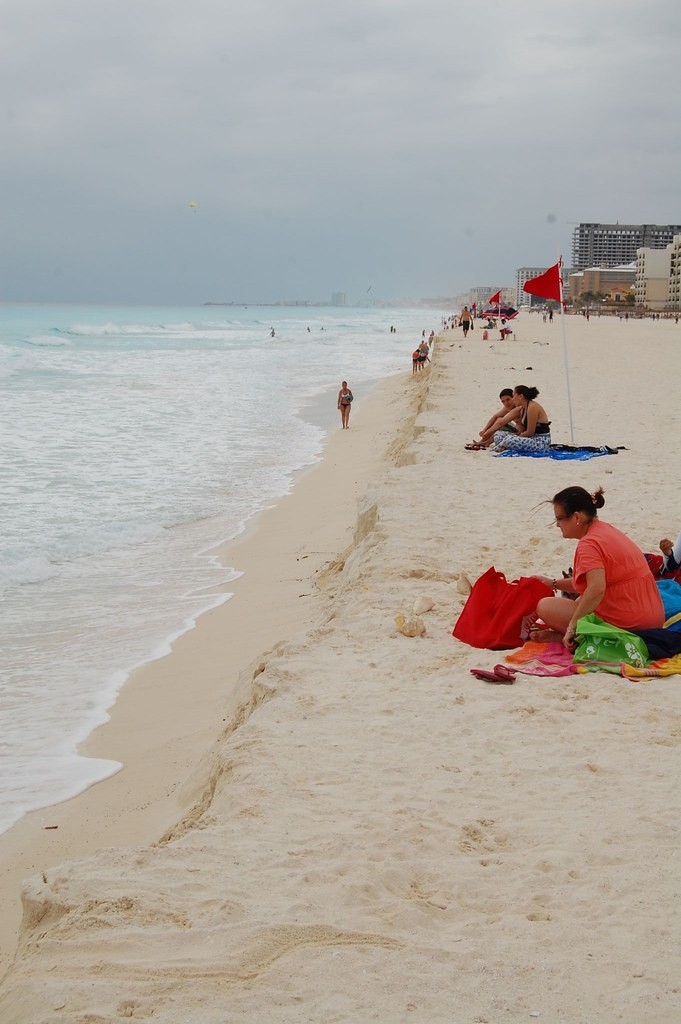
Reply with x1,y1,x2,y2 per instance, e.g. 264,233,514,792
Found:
556,513,573,521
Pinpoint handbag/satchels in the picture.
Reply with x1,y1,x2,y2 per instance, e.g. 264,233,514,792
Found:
453,565,555,651
573,612,650,668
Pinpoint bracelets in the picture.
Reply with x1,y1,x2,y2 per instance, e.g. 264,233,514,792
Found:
567,626,576,634
553,578,558,593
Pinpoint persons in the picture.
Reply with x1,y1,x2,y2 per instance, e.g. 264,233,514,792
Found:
412,330,434,374
530,486,665,654
441,301,678,341
337,380,353,429
659,529,681,572
472,385,552,453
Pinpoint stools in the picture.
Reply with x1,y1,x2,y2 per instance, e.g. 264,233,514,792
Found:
506,334,515,341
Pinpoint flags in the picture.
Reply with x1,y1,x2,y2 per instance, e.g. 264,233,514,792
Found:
489,292,500,305
523,263,561,302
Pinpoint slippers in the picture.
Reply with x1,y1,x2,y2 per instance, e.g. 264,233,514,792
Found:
470,669,504,682
494,664,516,680
466,444,486,451
